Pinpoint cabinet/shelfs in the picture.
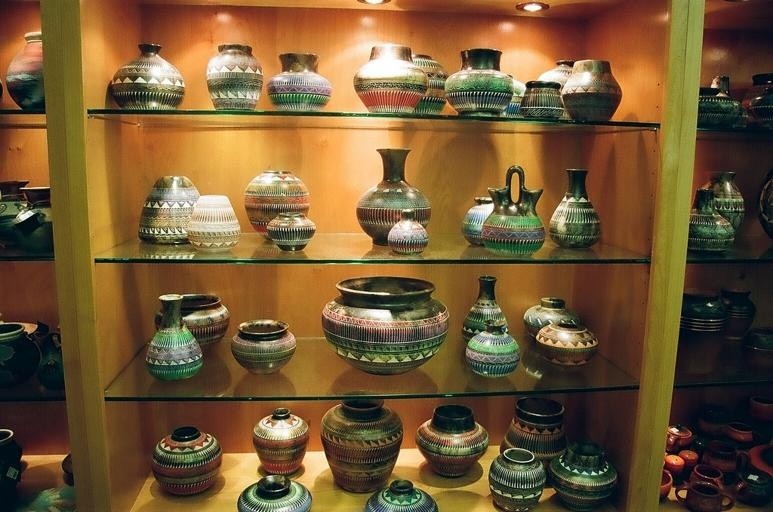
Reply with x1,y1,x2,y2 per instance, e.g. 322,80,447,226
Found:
0,2,773,511
